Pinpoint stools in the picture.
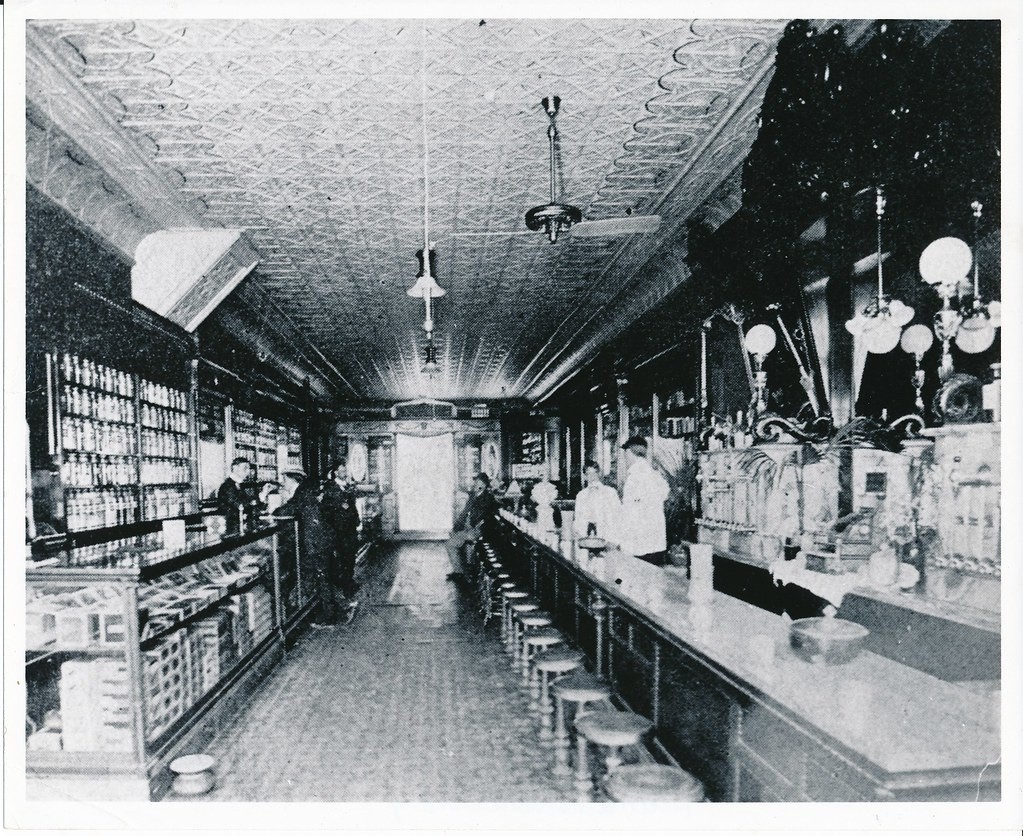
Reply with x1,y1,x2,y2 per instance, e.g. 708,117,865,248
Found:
600,762,705,802
549,675,616,791
575,711,655,794
474,540,565,740
529,649,588,775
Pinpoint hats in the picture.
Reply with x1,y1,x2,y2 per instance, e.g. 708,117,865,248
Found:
617,435,649,448
280,464,309,476
473,473,491,485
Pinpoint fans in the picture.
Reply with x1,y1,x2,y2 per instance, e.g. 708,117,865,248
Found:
448,95,661,244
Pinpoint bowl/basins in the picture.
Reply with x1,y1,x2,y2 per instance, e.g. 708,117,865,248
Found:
789,615,869,665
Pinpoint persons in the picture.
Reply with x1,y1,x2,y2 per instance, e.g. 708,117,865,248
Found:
320,461,360,593
621,436,670,568
445,472,500,583
271,463,358,631
571,460,624,551
217,457,258,537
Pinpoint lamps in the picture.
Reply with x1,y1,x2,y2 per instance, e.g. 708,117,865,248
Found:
901,323,933,414
932,198,1002,355
918,233,972,378
410,248,445,333
746,323,776,416
419,339,445,383
844,187,916,357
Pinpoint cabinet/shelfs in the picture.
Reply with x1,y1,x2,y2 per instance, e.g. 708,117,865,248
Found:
45,350,201,566
224,404,305,510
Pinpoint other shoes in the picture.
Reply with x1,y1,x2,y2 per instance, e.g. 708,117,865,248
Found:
312,602,360,634
446,570,463,581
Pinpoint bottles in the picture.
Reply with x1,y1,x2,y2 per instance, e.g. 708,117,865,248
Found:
229,409,302,486
58,351,196,562
